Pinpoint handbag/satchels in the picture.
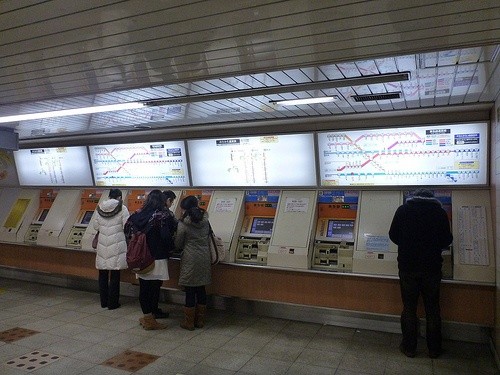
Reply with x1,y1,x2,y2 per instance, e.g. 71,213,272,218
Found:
207,219,225,266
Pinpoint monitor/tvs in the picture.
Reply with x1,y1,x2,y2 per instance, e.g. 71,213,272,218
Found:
38,209,50,221
250,217,274,234
326,220,355,239
81,211,94,224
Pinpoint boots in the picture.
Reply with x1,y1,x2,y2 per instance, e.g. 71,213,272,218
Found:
194,303,208,329
139,313,168,330
179,304,196,331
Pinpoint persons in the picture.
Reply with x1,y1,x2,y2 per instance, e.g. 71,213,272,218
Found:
91,188,130,310
388,188,454,359
169,195,212,331
124,190,180,330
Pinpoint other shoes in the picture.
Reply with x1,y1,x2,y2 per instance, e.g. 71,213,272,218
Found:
399,343,416,358
429,348,442,359
101,303,121,310
154,308,170,319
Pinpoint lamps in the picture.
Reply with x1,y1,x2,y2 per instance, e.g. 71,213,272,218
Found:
269,95,337,105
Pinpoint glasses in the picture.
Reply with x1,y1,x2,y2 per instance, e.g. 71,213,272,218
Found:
169,199,174,205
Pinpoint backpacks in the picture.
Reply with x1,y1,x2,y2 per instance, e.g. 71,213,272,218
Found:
125,218,156,275
